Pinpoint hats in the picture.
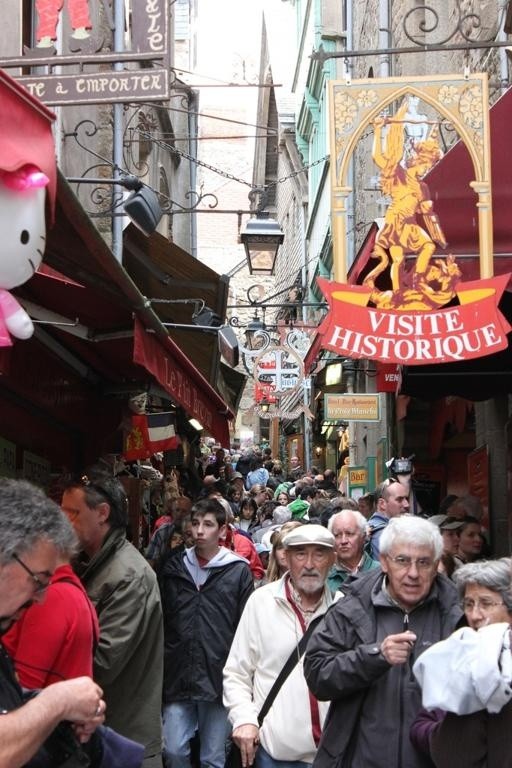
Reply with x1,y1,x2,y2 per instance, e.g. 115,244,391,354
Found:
229,472,245,483
428,515,464,530
281,524,335,547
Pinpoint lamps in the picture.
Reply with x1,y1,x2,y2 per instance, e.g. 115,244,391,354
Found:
159,203,287,278
64,171,166,239
258,394,271,414
147,295,220,336
225,302,270,352
308,356,347,373
323,362,377,388
161,320,239,367
314,444,324,460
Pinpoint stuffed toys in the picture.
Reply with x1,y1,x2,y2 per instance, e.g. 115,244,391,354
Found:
1,165,50,347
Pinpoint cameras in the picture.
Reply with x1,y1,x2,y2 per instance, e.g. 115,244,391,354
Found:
390,459,413,475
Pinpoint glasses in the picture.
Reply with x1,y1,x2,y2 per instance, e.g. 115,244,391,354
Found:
439,517,462,528
457,598,506,609
12,552,51,594
380,478,397,497
385,552,434,568
56,471,119,513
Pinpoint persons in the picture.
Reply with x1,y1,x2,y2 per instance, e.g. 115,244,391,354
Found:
0,437,512,768
370,117,446,307
